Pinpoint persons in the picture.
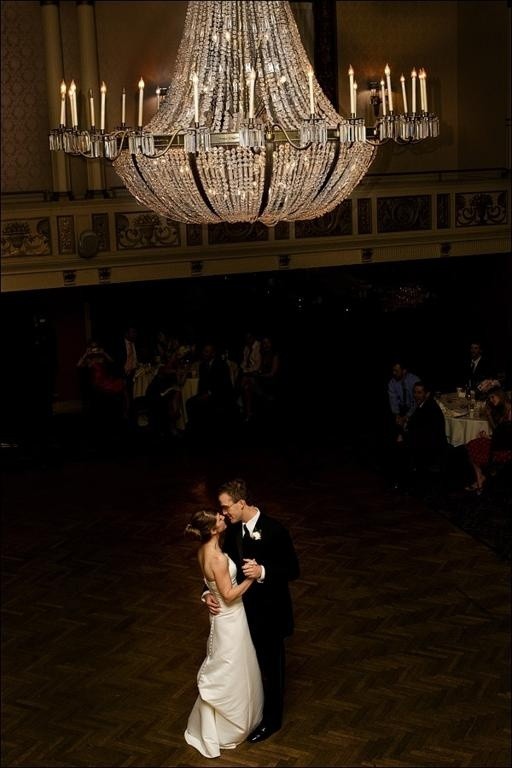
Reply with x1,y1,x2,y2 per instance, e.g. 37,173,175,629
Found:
385,357,424,433
184,507,265,760
458,338,498,384
74,325,282,447
396,381,445,495
462,386,512,496
200,476,302,742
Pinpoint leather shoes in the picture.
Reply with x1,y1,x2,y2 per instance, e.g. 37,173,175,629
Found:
252,716,281,742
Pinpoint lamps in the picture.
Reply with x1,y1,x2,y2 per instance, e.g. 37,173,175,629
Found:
40,0,440,236
